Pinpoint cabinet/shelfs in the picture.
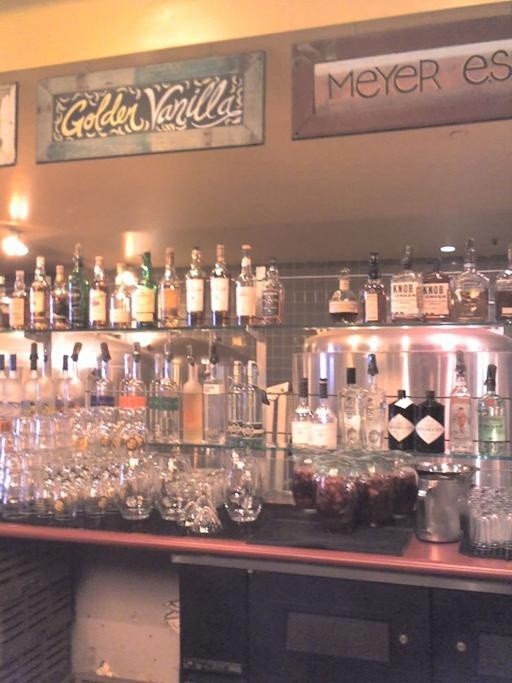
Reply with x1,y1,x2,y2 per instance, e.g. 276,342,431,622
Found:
178,560,512,683
0,252,512,506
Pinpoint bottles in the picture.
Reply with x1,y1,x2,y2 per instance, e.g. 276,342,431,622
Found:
292,353,506,457
1,244,283,331
328,240,511,324
1,341,258,446
282,446,420,535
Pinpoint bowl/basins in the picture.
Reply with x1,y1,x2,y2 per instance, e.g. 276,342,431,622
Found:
415,461,480,480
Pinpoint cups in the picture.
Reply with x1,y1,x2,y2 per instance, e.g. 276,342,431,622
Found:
0,408,262,534
465,485,512,551
415,477,461,543
